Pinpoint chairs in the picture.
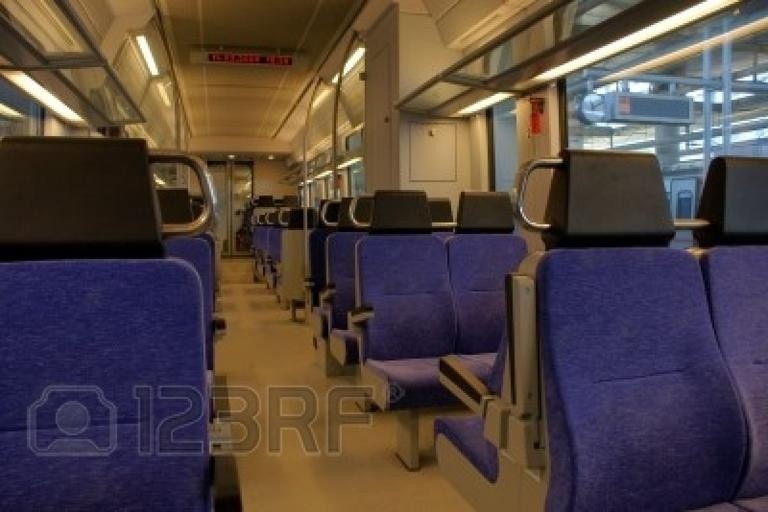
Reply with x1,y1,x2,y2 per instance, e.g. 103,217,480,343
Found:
443,188,531,399
693,148,767,512
511,148,749,508
0,135,242,511
431,249,541,512
250,193,454,376
347,186,493,471
